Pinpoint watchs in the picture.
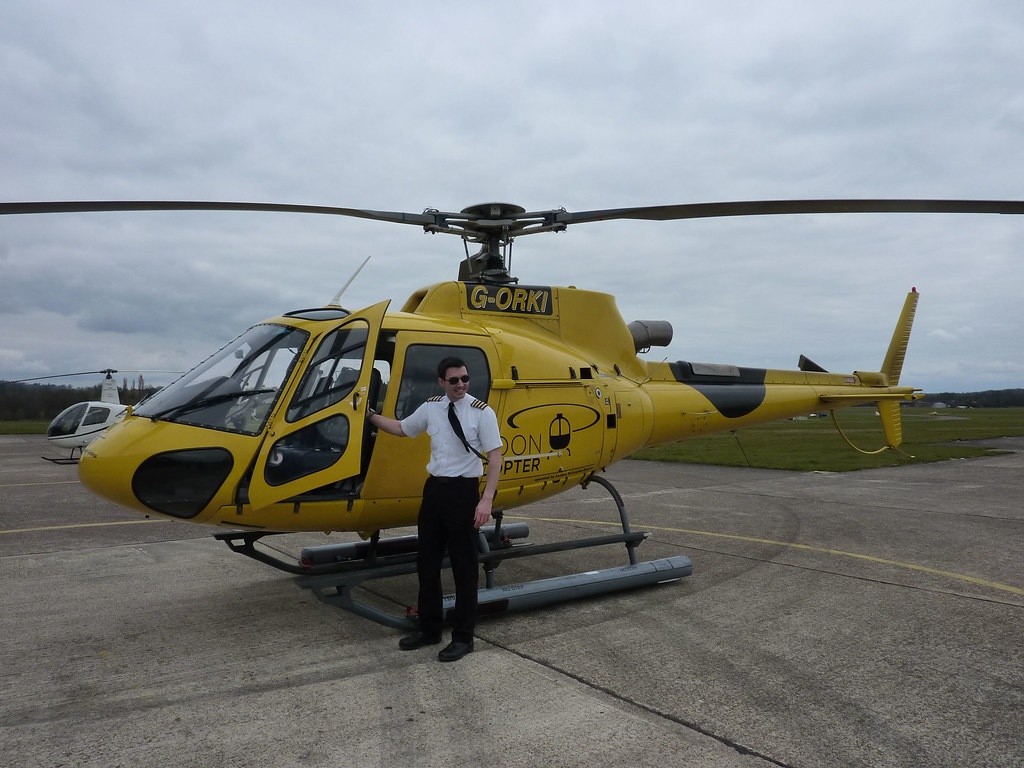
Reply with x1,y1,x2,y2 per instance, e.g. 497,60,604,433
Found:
367,408,376,420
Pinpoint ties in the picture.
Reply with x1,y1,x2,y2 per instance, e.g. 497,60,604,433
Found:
448,402,490,463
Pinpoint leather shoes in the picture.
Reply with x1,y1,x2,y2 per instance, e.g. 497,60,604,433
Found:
439,640,474,661
399,625,442,651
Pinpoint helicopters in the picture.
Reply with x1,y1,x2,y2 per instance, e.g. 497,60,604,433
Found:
0,194,1024,631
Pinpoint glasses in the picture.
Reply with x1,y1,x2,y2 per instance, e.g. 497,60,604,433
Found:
445,375,470,385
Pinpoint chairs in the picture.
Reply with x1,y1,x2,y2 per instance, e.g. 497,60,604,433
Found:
333,367,381,438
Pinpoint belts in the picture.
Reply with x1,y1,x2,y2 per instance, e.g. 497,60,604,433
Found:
430,475,479,483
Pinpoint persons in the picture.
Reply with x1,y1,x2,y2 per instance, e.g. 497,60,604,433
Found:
362,357,504,661
